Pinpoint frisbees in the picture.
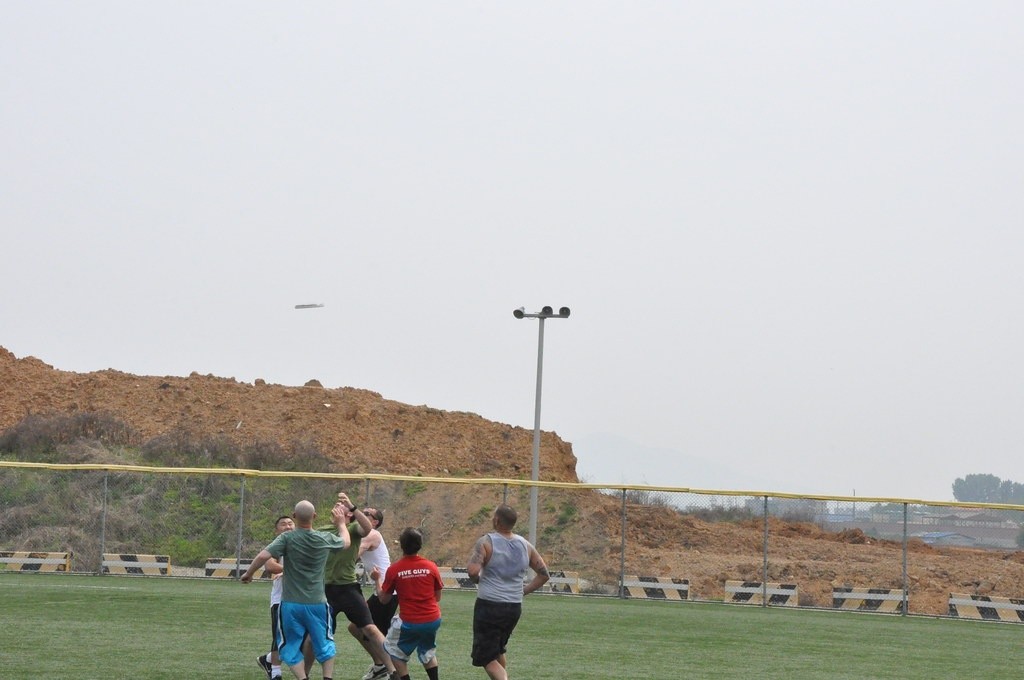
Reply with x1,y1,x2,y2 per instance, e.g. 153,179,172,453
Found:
295,304,318,309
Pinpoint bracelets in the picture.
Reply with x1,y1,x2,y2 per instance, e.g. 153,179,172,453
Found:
349,503,357,512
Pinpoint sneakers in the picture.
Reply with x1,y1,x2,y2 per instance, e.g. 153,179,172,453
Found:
257,655,282,680
362,664,387,680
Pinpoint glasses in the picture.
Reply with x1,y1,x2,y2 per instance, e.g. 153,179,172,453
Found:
362,511,376,521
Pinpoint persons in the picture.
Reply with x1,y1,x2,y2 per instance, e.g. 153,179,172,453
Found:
467,505,551,680
348,506,398,680
257,516,296,680
239,500,351,679
301,492,396,680
369,526,444,680
